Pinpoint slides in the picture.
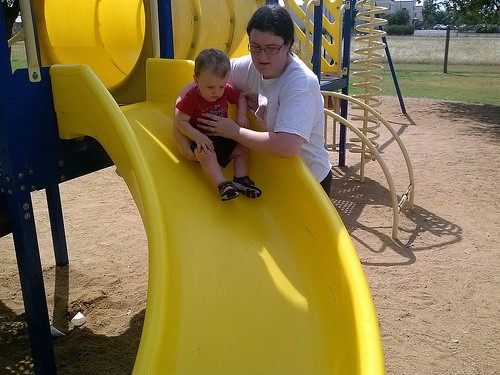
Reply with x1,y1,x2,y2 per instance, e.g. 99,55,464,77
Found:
51,57,387,375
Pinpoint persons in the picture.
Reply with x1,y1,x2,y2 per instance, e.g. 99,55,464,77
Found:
175,5,333,198
177,48,262,201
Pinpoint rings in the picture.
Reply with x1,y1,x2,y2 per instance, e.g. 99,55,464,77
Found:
212,128,215,132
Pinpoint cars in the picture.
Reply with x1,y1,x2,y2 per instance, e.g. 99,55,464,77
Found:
448,24,472,30
432,24,447,30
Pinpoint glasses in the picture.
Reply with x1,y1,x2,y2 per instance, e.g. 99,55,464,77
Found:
247,42,285,55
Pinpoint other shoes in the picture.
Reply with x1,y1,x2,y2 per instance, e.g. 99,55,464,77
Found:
232,176,262,199
218,181,239,201
